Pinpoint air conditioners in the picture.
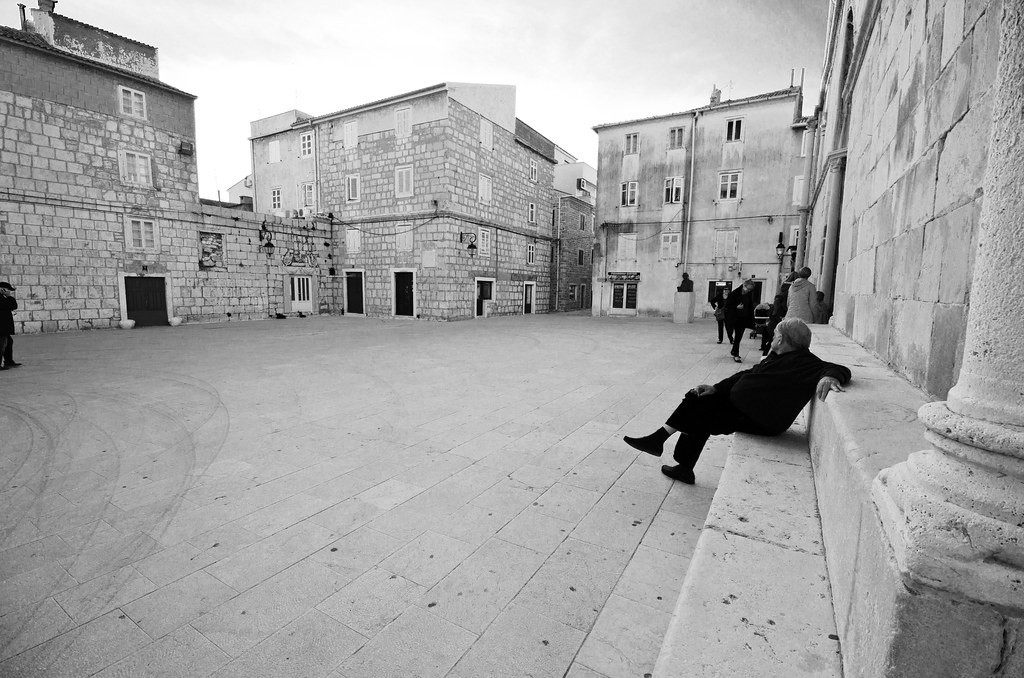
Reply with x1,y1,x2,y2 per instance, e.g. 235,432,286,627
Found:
298,208,309,217
577,179,586,189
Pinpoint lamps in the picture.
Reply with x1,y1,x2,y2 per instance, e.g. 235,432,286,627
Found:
259,220,275,257
776,242,796,259
460,232,477,258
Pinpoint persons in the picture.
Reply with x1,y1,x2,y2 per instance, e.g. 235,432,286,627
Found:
815,291,831,322
0,281,23,370
761,273,799,356
709,286,738,345
784,267,820,324
622,318,852,485
725,280,760,363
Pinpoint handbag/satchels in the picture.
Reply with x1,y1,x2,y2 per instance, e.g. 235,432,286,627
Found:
713,308,725,320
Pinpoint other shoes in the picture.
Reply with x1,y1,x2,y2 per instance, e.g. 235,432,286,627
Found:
623,436,663,456
763,352,767,356
717,341,722,344
730,341,734,344
662,465,695,484
759,347,764,350
733,356,741,363
731,351,741,358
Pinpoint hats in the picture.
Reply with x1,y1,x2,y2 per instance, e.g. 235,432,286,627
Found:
722,285,731,290
777,319,811,348
